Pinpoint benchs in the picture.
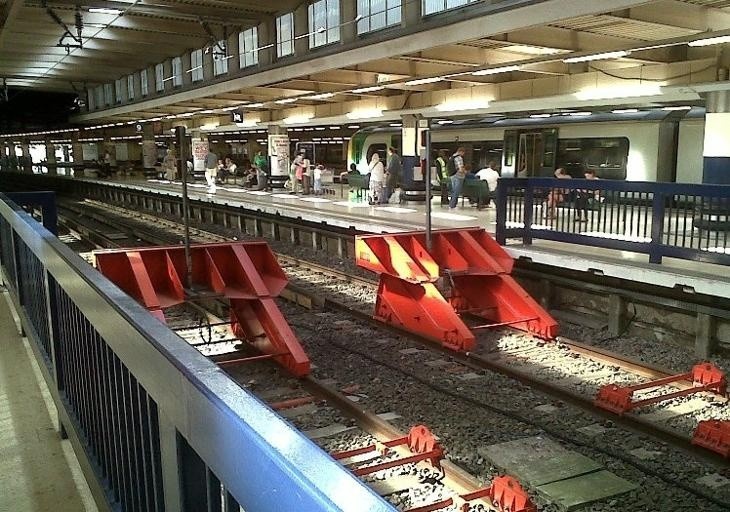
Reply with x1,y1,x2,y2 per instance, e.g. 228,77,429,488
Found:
341,173,370,200
440,178,497,210
541,190,607,222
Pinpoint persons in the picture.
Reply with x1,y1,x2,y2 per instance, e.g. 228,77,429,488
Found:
163,149,193,182
204,148,268,188
348,146,400,205
545,168,600,221
92,149,118,178
287,151,326,195
436,146,498,211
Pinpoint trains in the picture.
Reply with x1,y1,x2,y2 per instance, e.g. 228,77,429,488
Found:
347,108,705,207
